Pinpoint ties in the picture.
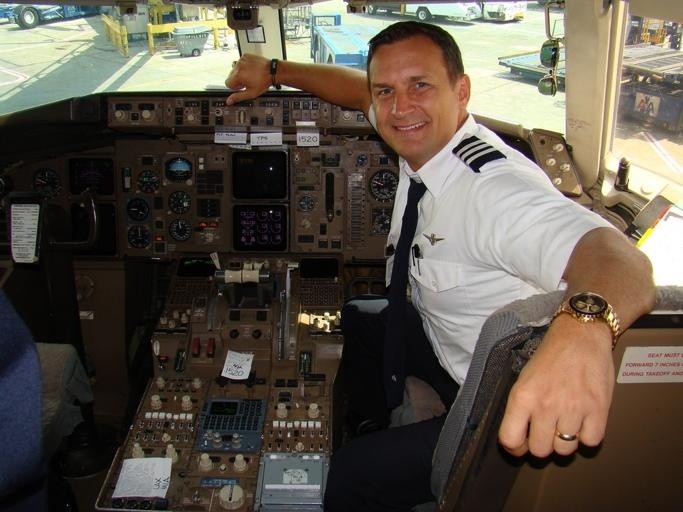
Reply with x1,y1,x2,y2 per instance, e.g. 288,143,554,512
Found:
389,178,427,305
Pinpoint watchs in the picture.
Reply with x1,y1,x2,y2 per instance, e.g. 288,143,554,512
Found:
546,289,623,352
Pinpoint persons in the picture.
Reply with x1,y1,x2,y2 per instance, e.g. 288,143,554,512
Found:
221,18,658,512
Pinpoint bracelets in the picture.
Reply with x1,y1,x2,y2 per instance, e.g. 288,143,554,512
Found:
269,57,284,91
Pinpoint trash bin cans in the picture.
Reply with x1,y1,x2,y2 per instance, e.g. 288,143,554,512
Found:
172,26,211,58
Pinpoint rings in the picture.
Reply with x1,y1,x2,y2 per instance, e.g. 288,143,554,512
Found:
555,424,580,445
231,60,237,68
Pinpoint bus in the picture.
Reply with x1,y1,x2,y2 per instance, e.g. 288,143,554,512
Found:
0,3,108,30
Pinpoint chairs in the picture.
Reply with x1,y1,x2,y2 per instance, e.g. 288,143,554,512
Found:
429,285,683,512
0,294,93,512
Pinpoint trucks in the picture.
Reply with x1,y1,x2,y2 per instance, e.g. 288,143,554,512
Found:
366,0,483,24
